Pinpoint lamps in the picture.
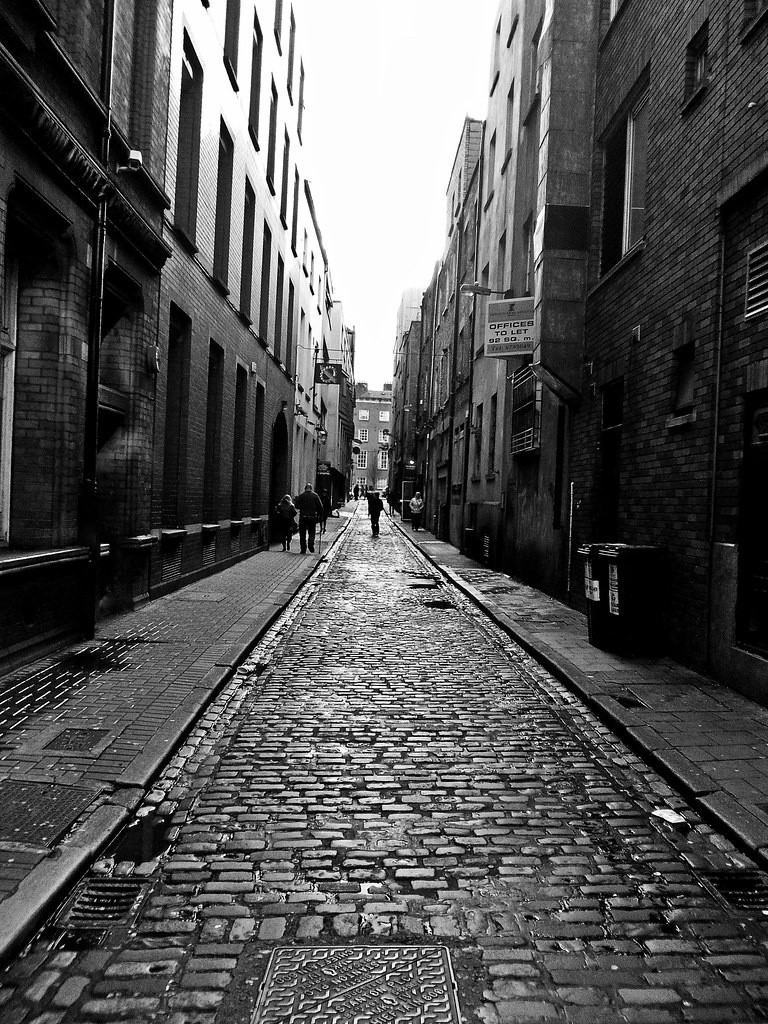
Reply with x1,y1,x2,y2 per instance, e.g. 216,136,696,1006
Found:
459,281,513,299
282,399,288,411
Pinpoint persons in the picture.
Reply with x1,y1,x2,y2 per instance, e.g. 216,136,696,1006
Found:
354,484,376,500
277,495,297,551
387,492,398,515
317,489,332,533
409,492,424,531
294,484,322,554
368,492,383,537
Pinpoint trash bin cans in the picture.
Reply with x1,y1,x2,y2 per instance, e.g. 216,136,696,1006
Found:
580,542,668,655
